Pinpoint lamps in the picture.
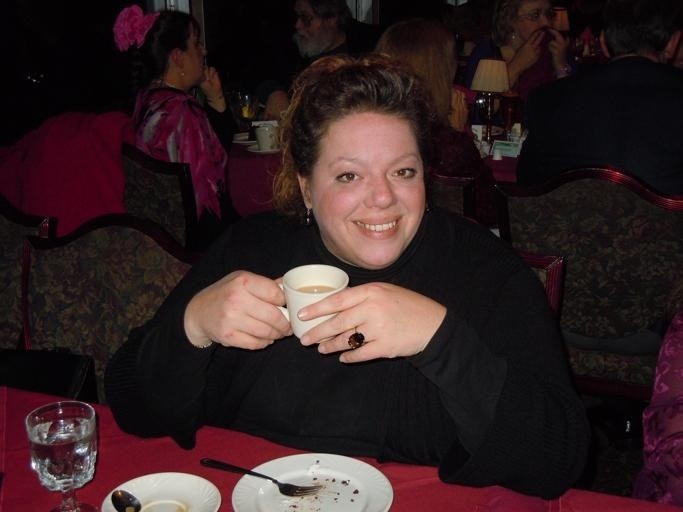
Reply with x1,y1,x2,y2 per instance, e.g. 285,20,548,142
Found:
469,59,511,147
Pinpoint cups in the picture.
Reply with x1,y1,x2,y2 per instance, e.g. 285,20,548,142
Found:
238,94,258,141
492,149,502,161
254,124,279,151
470,124,487,143
273,264,350,343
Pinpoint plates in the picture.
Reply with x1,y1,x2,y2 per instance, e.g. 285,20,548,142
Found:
490,127,504,136
99,471,223,512
230,131,256,146
248,143,282,155
230,452,394,512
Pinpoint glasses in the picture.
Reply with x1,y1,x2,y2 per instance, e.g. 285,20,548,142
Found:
515,8,557,22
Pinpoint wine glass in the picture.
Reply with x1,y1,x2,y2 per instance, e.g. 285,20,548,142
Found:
23,399,98,512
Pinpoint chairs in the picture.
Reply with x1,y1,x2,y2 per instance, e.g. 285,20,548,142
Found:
0,193,58,351
515,251,566,316
19,210,208,405
118,138,199,250
424,171,474,222
480,162,683,397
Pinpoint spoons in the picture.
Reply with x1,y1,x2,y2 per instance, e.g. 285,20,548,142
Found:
110,489,141,512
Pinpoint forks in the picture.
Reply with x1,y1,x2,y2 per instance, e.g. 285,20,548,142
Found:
200,457,323,496
